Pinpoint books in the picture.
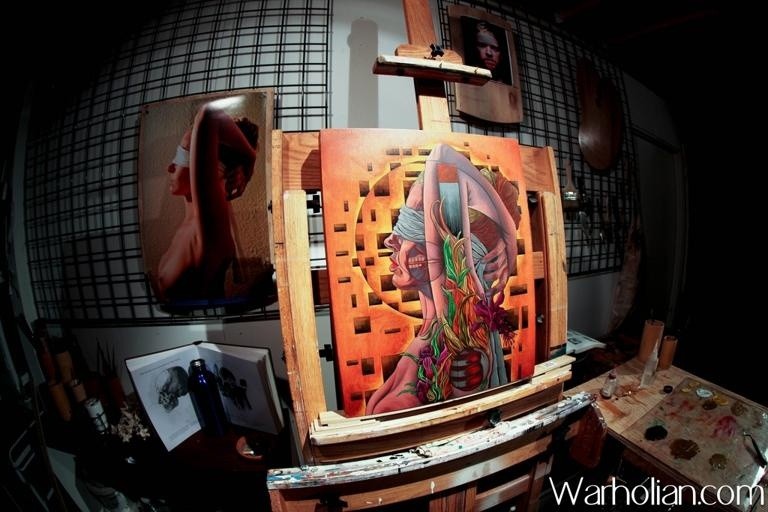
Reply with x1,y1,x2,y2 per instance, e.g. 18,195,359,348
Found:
124,341,286,454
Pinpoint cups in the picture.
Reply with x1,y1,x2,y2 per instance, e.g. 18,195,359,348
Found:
46,378,87,424
660,337,678,369
639,320,665,364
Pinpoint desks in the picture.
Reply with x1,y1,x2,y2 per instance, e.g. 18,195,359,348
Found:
111,390,288,512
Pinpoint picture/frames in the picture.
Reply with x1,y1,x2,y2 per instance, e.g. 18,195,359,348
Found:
131,84,278,316
446,5,522,124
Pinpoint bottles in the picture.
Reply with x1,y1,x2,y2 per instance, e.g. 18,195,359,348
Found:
189,359,228,438
639,341,660,388
601,368,618,398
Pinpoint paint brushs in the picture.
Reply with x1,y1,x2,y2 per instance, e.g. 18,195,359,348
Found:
95,336,117,379
71,368,78,386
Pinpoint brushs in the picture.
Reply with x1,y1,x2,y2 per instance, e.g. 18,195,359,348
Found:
605,191,613,244
562,158,595,216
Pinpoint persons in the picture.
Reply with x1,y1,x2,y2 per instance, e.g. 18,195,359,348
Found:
471,24,505,70
153,103,261,303
361,139,521,418
